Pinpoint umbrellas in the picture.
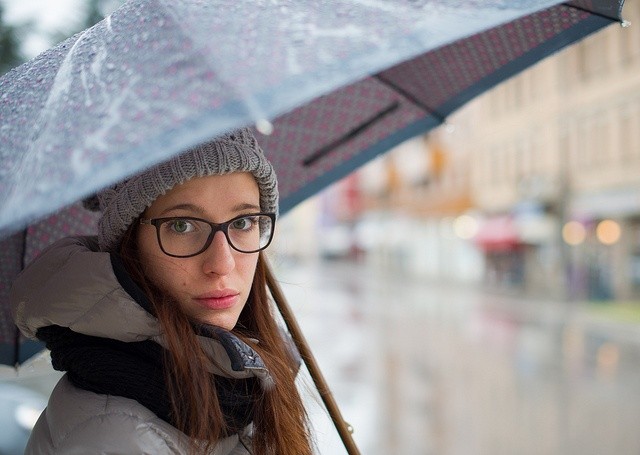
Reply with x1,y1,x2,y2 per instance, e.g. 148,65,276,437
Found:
0,1,631,453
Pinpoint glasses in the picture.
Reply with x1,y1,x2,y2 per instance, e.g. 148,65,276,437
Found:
137,212,276,259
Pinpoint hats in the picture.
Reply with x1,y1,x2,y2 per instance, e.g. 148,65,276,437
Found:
97,125,279,253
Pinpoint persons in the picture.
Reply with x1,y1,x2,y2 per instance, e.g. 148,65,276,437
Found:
10,126,315,454
